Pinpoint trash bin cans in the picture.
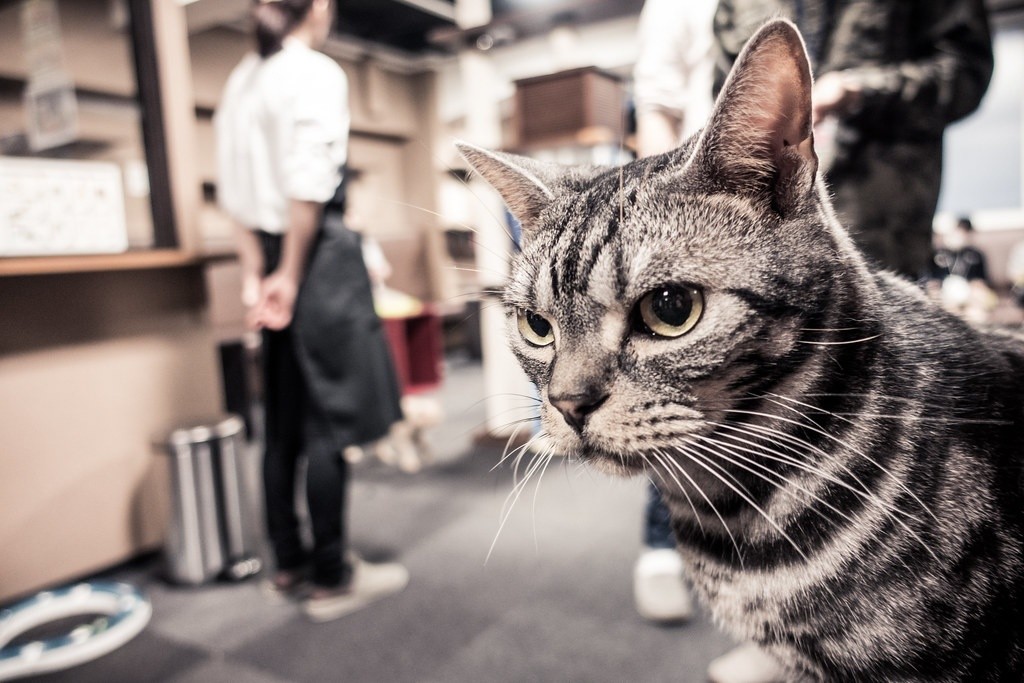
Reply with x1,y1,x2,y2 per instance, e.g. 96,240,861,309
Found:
150,413,248,587
479,286,544,441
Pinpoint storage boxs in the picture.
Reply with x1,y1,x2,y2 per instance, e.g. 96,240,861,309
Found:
513,64,626,144
385,310,445,395
0,156,130,255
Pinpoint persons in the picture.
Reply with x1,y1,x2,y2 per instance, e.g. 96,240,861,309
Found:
623,1,994,623
221,0,410,620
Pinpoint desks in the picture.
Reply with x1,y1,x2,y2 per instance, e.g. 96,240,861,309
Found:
0,245,243,607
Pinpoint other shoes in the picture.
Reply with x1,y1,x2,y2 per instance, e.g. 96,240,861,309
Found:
632,548,695,624
305,550,410,623
262,564,311,599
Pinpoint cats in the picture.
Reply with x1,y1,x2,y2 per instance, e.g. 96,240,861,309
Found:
453,18,1024,683
